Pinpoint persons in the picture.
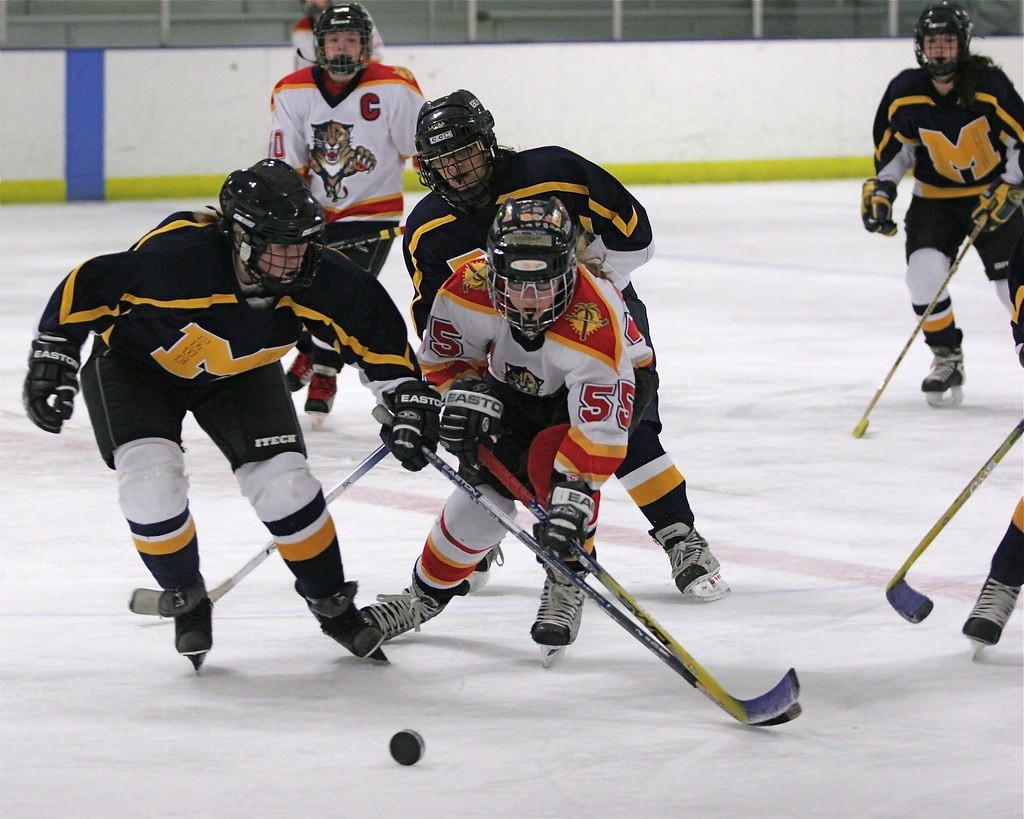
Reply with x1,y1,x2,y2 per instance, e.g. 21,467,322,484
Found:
962,496,1024,646
268,0,426,413
21,158,439,658
861,0,1024,393
357,90,722,646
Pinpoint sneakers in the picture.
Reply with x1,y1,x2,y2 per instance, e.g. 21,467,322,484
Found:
163,572,214,677
529,545,597,669
647,516,732,602
295,578,388,663
361,554,470,644
468,541,505,594
284,352,337,430
921,327,966,407
962,574,1022,660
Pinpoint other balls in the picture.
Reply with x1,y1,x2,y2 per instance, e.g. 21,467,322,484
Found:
388,728,424,765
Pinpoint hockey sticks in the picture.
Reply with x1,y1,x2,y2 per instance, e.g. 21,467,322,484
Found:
884,418,1024,623
326,223,404,251
855,215,990,441
373,401,803,727
128,443,388,617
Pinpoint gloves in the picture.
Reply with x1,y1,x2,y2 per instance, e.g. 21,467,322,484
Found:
379,380,443,473
532,466,595,557
439,379,503,476
23,331,81,434
971,177,1024,232
861,178,898,236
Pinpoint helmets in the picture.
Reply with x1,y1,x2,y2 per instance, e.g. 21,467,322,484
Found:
219,158,325,296
915,2,970,84
414,89,504,221
486,196,578,337
313,3,373,76
